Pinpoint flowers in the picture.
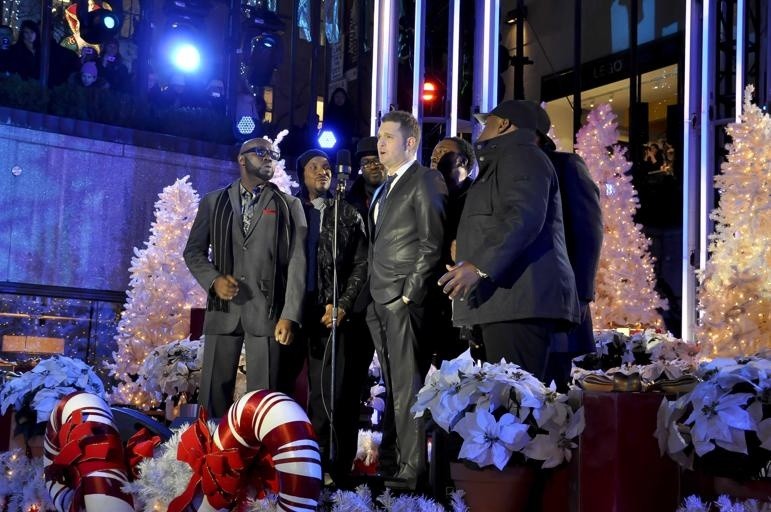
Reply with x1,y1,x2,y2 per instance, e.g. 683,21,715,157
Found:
571,331,771,509
1,355,109,509
313,351,588,510
108,339,252,509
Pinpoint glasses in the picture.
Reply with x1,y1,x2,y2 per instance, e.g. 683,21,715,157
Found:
242,146,280,161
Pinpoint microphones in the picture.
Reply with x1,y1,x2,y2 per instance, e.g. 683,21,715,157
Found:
335,149,351,192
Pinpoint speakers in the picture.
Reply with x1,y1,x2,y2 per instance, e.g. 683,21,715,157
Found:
190,308,206,340
109,406,175,445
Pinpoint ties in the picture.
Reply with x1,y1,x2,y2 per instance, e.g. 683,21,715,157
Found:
374,174,398,239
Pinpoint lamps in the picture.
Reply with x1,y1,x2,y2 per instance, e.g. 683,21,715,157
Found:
75,0,127,47
241,1,288,90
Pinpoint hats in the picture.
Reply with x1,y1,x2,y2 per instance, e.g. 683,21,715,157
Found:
354,136,379,159
472,100,556,151
296,149,328,181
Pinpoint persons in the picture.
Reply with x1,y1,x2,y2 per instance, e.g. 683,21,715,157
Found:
183,138,308,425
282,149,367,492
